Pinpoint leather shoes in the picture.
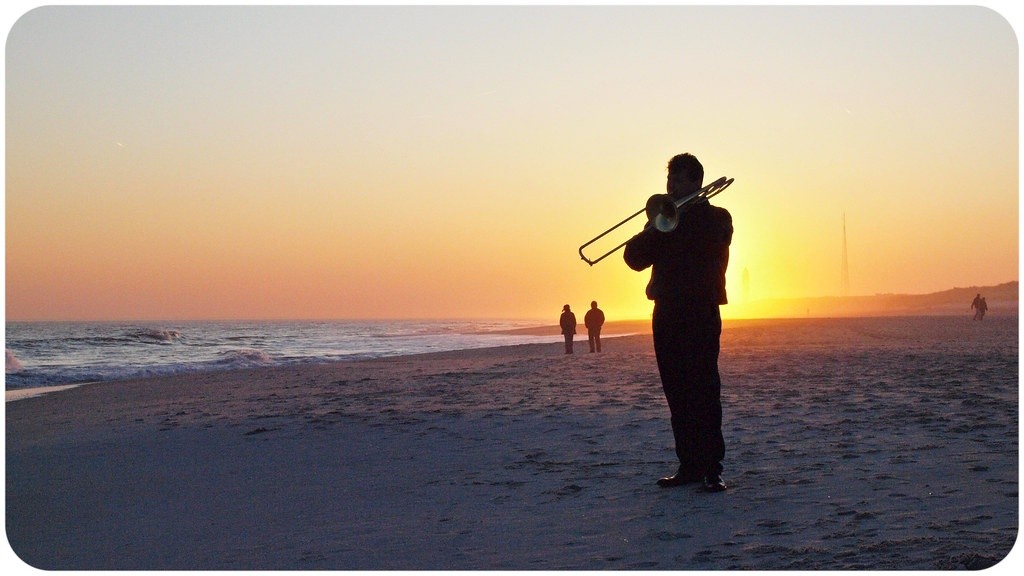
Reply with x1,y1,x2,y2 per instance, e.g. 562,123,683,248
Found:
656,470,699,487
704,473,727,492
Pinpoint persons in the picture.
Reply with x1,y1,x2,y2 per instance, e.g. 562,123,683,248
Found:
971,294,981,320
623,151,735,489
559,304,577,354
978,297,988,320
584,301,606,353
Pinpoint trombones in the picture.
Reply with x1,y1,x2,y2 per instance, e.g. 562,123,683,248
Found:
579,176,736,266
